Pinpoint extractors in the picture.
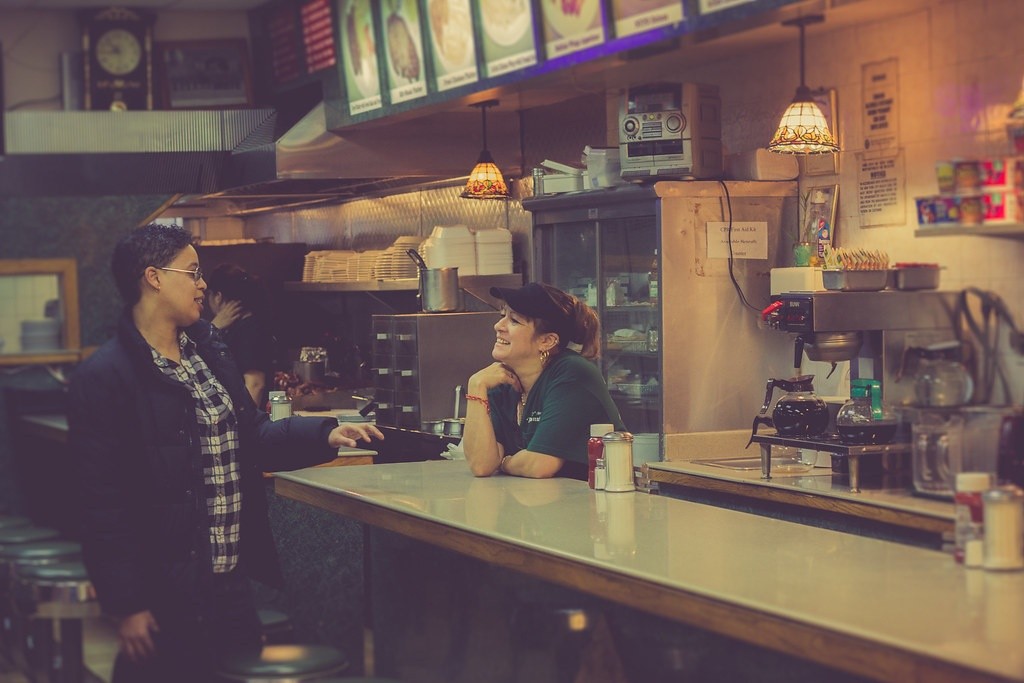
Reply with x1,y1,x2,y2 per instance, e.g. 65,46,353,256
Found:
224,93,524,209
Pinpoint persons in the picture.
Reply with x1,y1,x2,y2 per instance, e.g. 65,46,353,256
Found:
206,263,268,409
463,280,638,486
66,221,384,683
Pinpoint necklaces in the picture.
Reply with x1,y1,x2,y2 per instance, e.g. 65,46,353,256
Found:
521,393,526,406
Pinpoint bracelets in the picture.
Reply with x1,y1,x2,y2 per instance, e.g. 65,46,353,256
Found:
501,455,511,474
466,394,491,416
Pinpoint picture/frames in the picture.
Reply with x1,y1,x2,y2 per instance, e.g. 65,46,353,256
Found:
153,37,256,111
802,184,839,248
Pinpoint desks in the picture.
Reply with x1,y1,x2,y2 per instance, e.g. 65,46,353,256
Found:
273,459,1024,683
642,455,957,538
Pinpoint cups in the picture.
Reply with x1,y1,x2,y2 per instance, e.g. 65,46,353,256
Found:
792,242,816,266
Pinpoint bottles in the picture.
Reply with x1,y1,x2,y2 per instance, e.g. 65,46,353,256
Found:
648,249,658,307
648,326,658,353
586,423,635,492
533,168,545,197
956,472,1024,572
807,191,832,267
270,396,292,422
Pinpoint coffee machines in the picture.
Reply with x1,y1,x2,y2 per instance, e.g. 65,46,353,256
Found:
753,290,965,494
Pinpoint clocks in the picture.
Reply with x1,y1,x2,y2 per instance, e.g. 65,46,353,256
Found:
78,5,158,111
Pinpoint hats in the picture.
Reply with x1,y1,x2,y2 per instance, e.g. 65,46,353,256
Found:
489,281,575,339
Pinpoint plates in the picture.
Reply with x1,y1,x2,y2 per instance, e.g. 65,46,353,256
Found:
348,0,601,99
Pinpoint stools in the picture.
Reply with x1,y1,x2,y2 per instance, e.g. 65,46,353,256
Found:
0,515,349,683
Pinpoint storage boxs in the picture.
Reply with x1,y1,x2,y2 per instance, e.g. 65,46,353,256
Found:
540,144,619,194
815,264,949,291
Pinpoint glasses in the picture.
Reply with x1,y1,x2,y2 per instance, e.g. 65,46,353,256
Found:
139,267,208,282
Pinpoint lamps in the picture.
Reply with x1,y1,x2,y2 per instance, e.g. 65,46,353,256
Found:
766,10,847,154
459,98,511,199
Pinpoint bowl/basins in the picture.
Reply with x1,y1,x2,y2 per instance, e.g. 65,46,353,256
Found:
302,224,514,284
422,416,466,435
913,157,1024,225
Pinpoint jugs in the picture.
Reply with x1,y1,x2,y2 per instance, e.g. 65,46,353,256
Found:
758,339,1014,499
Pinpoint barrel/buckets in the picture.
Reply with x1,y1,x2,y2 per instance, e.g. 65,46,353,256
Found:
417,265,459,313
